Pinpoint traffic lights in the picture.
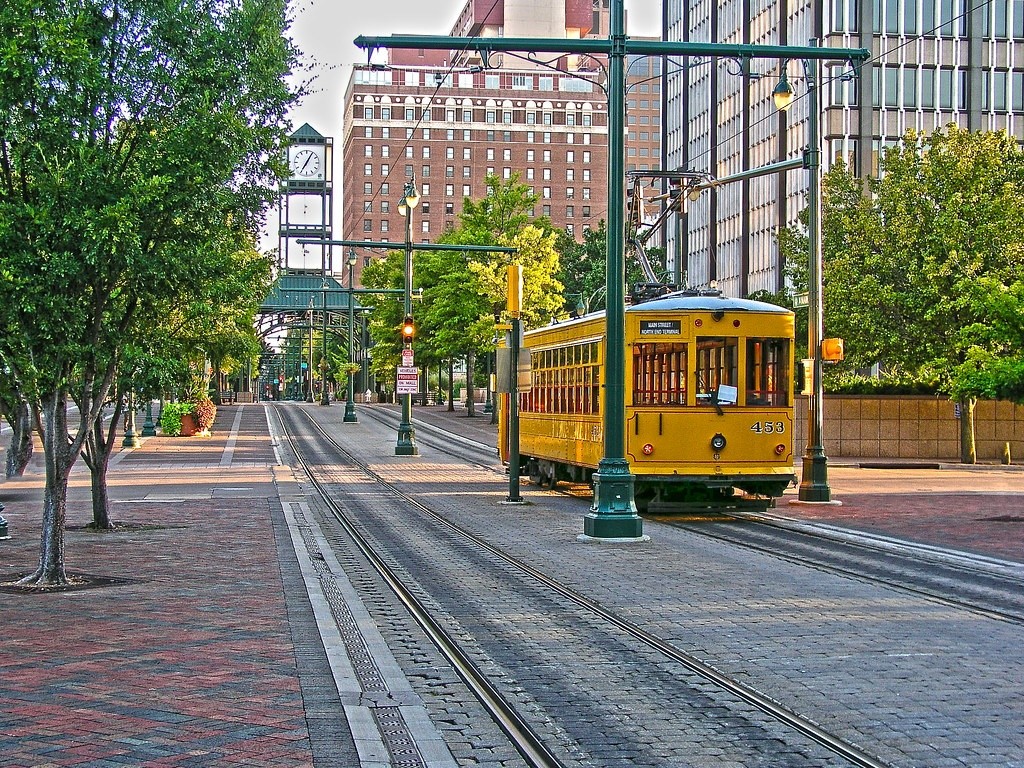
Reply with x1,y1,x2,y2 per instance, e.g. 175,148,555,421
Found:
404,316,414,344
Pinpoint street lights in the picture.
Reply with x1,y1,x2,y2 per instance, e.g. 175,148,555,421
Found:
396,171,421,456
687,37,845,505
306,276,334,407
345,239,360,424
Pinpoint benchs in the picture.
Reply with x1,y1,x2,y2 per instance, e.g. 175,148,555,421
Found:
397,391,439,406
219,389,236,405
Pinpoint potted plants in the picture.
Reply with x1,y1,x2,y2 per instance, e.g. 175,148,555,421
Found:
160,374,218,437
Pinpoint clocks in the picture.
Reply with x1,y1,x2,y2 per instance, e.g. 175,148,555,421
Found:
293,149,321,177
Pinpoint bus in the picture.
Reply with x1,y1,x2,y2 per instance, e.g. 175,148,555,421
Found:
496,182,796,515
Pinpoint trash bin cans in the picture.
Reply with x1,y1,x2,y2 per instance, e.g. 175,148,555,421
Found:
253,394,259,403
